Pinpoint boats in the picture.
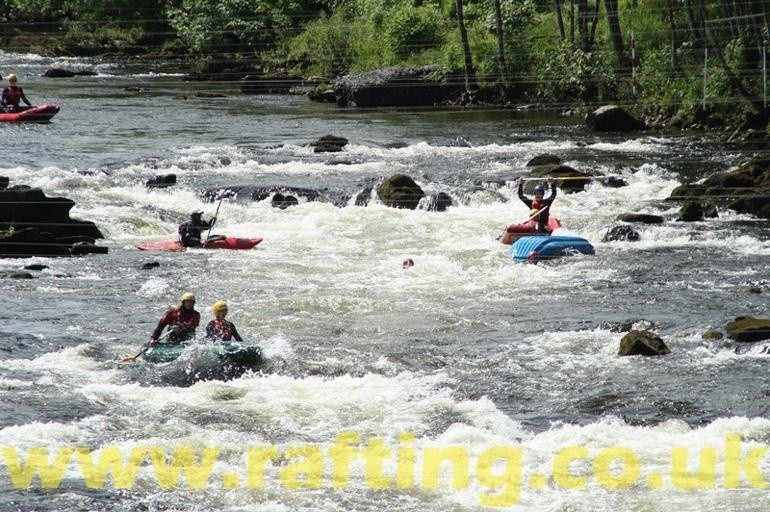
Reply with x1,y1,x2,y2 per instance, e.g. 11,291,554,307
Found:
139,339,264,367
507,234,597,263
0,102,62,124
501,215,562,245
133,234,266,254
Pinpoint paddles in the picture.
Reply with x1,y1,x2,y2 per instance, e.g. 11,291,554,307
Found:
122,330,174,362
514,173,621,182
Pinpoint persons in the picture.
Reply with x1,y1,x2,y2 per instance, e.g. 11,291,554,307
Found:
528,251,539,265
206,301,243,342
518,177,556,234
151,293,200,344
2,73,32,111
181,209,216,248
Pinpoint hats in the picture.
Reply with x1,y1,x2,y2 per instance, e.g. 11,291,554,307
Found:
190,208,204,216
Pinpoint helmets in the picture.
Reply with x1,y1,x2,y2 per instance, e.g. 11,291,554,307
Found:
180,293,195,301
528,252,539,260
533,186,544,193
214,301,227,312
7,73,17,80
402,257,414,266
528,209,540,217
